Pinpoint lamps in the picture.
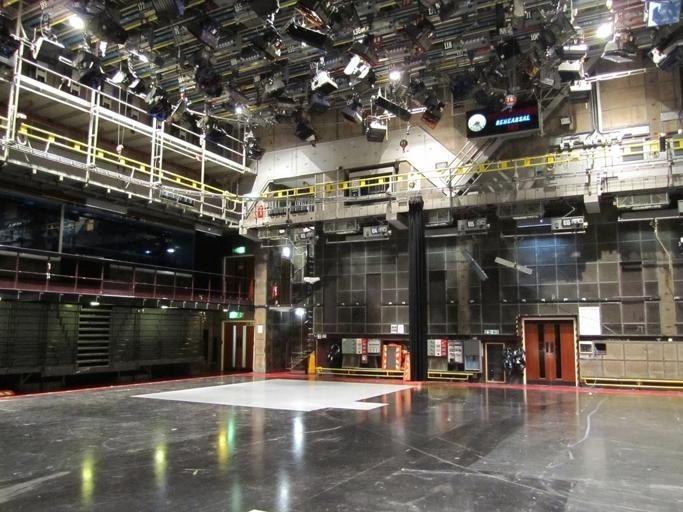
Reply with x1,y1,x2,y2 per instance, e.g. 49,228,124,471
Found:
84,199,128,215
194,223,222,237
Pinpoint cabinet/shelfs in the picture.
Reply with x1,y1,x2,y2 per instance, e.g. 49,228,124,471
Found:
578,341,683,380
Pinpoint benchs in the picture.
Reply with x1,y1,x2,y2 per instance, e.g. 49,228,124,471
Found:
580,378,683,391
315,366,474,381
